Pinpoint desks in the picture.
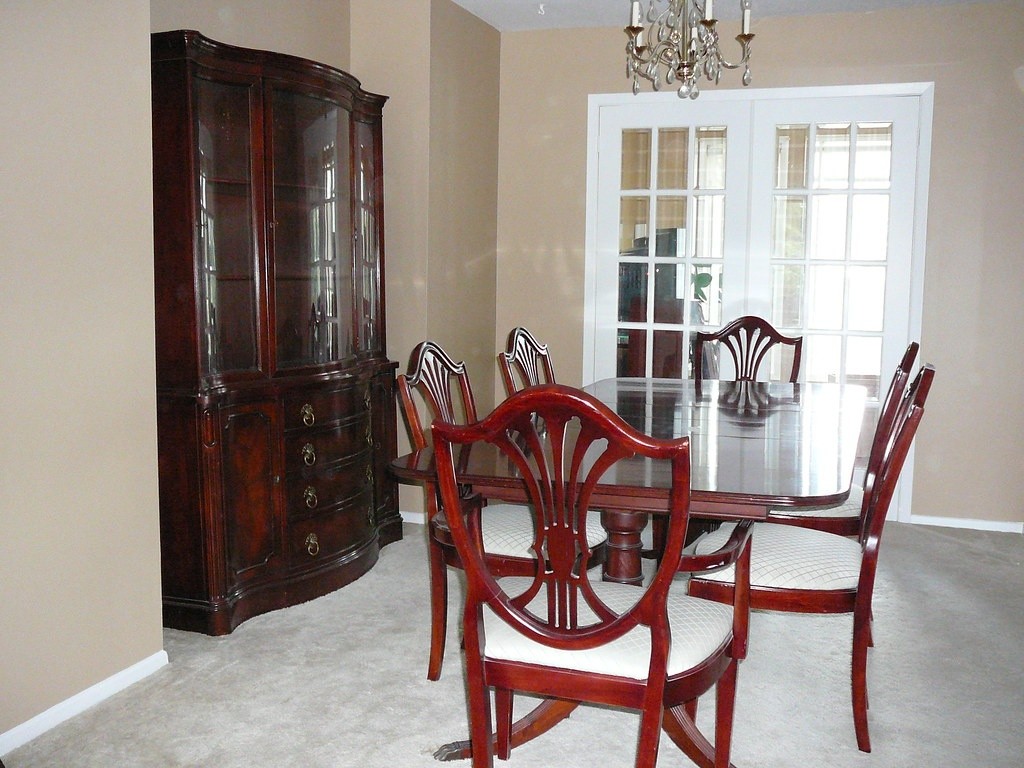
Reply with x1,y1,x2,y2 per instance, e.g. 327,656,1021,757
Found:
390,377,870,768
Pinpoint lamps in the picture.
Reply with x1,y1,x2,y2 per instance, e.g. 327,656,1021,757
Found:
626,0,756,102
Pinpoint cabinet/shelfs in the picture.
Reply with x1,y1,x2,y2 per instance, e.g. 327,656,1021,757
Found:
152,29,405,637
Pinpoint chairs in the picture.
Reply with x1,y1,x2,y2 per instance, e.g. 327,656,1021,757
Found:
684,361,937,741
432,386,752,768
498,327,554,390
394,341,608,677
691,316,801,381
768,340,920,539
